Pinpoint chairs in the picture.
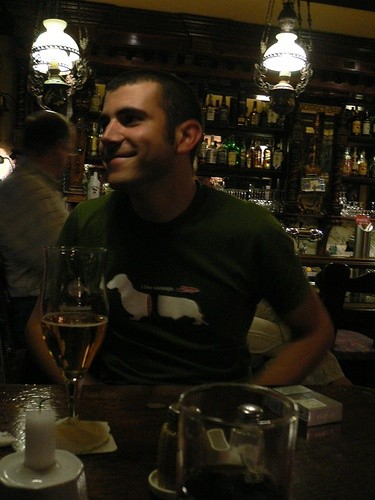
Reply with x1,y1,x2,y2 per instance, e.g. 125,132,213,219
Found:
312,263,375,389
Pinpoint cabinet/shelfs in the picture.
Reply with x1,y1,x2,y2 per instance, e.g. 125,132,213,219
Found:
59,52,375,311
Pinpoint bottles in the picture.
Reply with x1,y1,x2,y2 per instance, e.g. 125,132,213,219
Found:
81,165,108,202
156,402,204,490
88,122,104,157
197,91,375,178
89,86,101,112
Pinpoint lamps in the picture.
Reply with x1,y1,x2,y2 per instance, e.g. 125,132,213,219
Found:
257,0,314,109
26,1,91,108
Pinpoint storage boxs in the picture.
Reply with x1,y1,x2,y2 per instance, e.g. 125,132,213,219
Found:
272,385,343,427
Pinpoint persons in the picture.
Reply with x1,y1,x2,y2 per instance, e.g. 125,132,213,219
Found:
26,73,333,385
0,112,73,347
257,304,352,386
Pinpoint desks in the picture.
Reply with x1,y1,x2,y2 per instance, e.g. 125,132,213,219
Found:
0,380,375,500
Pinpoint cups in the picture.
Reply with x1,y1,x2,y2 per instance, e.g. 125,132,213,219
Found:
355,219,370,258
177,381,301,500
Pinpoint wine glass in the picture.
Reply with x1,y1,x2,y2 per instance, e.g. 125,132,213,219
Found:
338,191,375,218
37,244,113,454
